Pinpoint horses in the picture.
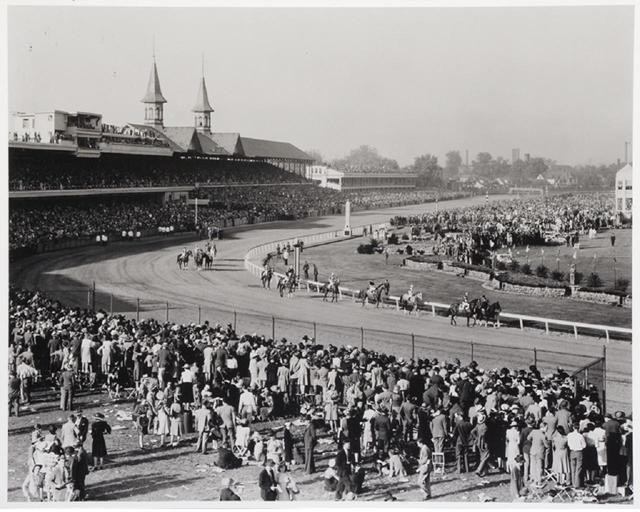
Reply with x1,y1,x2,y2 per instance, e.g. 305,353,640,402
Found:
277,272,297,299
176,249,193,270
358,279,390,309
399,292,425,314
260,267,274,289
475,301,502,328
195,246,202,270
318,278,341,302
444,299,482,327
202,250,214,270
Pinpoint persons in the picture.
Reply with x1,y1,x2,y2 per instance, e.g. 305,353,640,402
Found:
8,286,634,504
9,123,634,327
